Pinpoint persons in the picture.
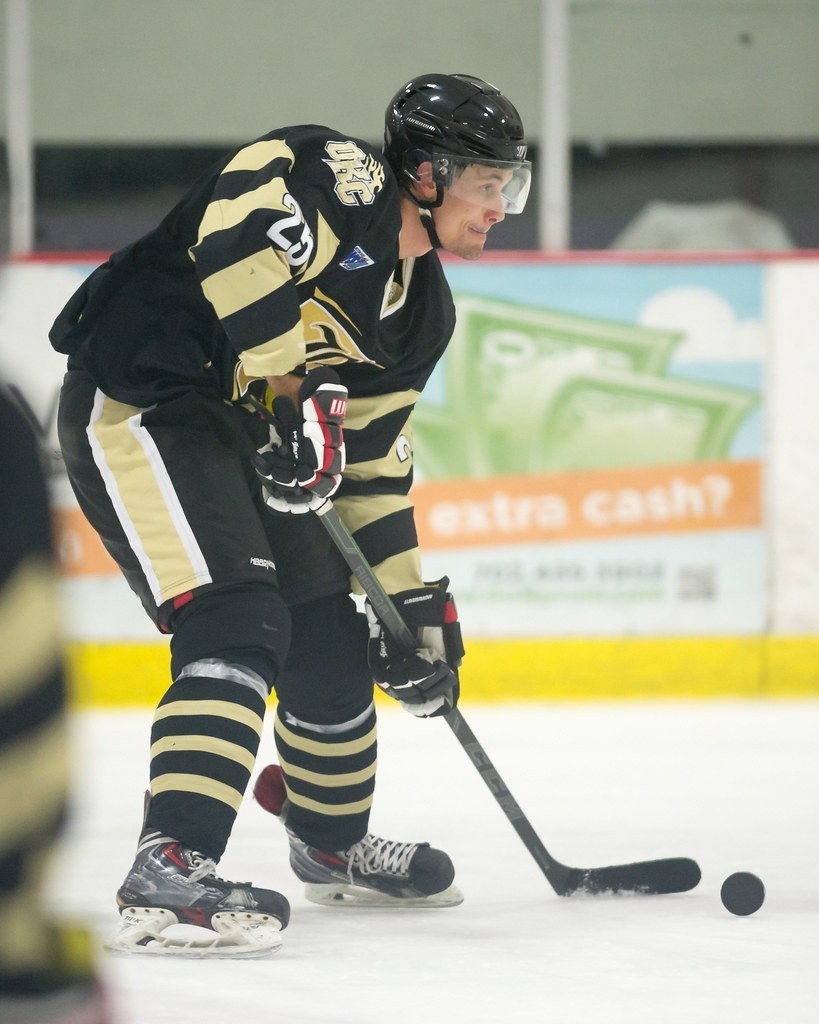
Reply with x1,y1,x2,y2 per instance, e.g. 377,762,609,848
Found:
48,74,531,958
0,380,120,1024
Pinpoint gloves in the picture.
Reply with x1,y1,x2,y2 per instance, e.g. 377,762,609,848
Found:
252,367,349,514
365,575,465,719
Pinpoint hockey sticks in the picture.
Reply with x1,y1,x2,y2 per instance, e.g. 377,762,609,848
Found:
272,393,702,896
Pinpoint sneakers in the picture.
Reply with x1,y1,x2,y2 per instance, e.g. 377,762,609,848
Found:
114,790,292,954
283,801,464,908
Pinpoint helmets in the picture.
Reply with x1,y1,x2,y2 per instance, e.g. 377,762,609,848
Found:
383,74,527,187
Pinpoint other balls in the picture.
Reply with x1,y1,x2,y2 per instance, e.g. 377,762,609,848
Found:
719,872,766,917
